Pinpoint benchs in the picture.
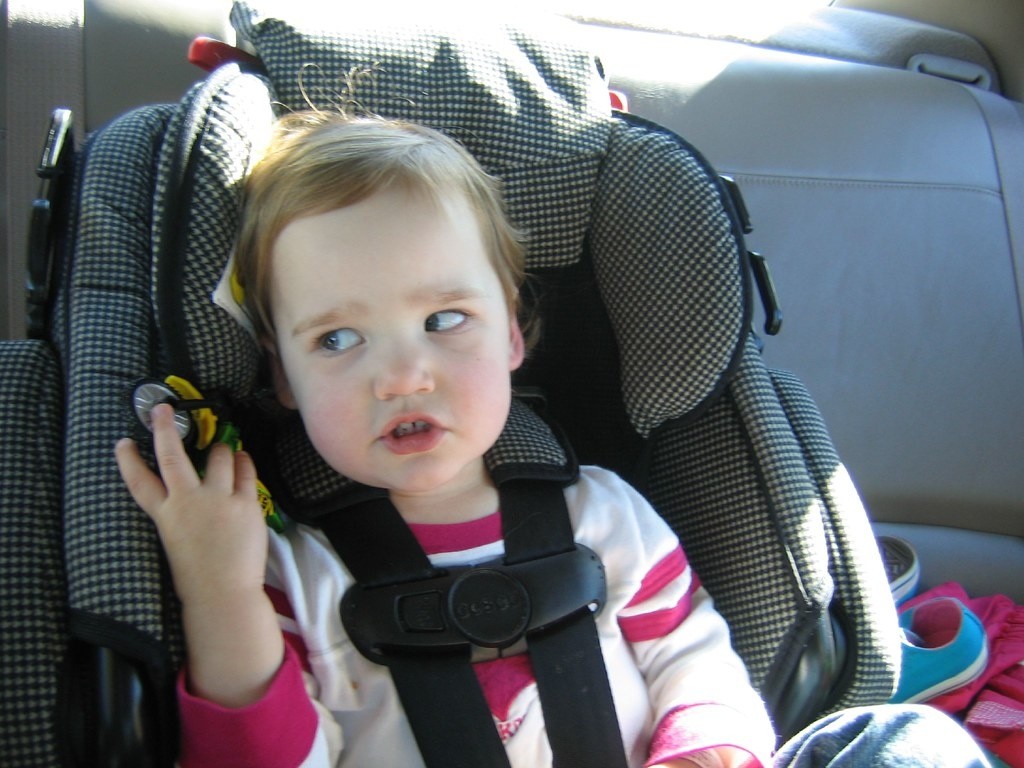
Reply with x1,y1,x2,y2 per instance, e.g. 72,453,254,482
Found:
1,2,1023,629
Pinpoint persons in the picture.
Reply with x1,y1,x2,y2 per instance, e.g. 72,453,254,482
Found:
116,113,989,768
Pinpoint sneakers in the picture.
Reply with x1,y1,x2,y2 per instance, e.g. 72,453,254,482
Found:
874,535,920,609
887,597,988,704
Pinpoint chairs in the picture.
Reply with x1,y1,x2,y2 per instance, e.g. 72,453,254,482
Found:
0,1,904,767
